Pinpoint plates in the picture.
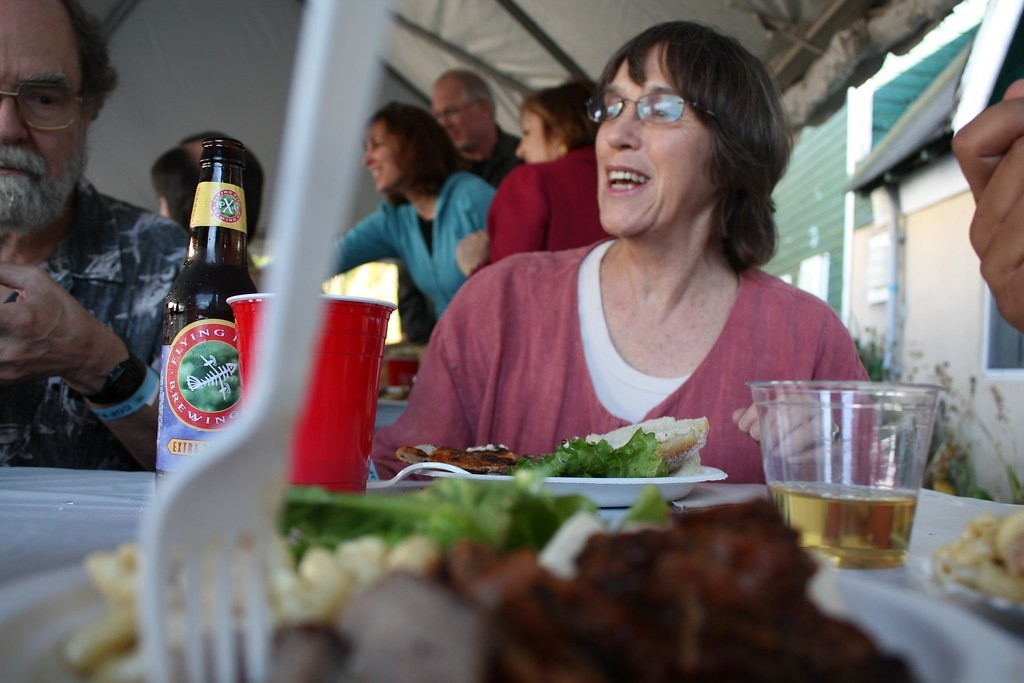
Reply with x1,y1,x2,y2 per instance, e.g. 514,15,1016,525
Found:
923,561,1024,628
416,465,729,509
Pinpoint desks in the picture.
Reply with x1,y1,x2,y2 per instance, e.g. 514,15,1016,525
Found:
0,463,1024,683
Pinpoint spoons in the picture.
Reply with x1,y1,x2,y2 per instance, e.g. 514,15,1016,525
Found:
366,461,470,500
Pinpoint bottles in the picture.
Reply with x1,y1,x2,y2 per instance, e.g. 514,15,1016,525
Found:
154,138,257,488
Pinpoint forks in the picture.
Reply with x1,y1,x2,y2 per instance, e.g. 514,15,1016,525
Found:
139,0,396,683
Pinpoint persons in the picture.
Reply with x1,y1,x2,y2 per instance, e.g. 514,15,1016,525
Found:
429,68,524,188
151,129,264,245
1,2,193,472
248,102,497,322
487,80,616,264
370,19,895,482
951,78,1024,334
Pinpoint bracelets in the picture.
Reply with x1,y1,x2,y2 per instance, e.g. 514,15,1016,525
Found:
92,366,157,422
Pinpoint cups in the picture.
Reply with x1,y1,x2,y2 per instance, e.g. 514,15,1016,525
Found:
222,293,398,498
744,380,949,569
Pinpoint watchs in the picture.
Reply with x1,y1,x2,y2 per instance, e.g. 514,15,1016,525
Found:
85,353,147,405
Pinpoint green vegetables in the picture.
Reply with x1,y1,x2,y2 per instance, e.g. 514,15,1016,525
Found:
277,426,673,549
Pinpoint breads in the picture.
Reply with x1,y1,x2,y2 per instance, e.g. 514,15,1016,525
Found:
582,415,709,467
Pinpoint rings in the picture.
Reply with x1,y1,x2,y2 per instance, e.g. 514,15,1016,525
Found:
832,420,841,440
3,290,20,304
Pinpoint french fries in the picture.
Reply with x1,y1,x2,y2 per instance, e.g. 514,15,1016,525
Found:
941,506,1024,607
59,527,439,683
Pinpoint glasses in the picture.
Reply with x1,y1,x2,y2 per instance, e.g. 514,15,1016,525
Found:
433,101,473,118
585,89,721,125
0,82,88,131
